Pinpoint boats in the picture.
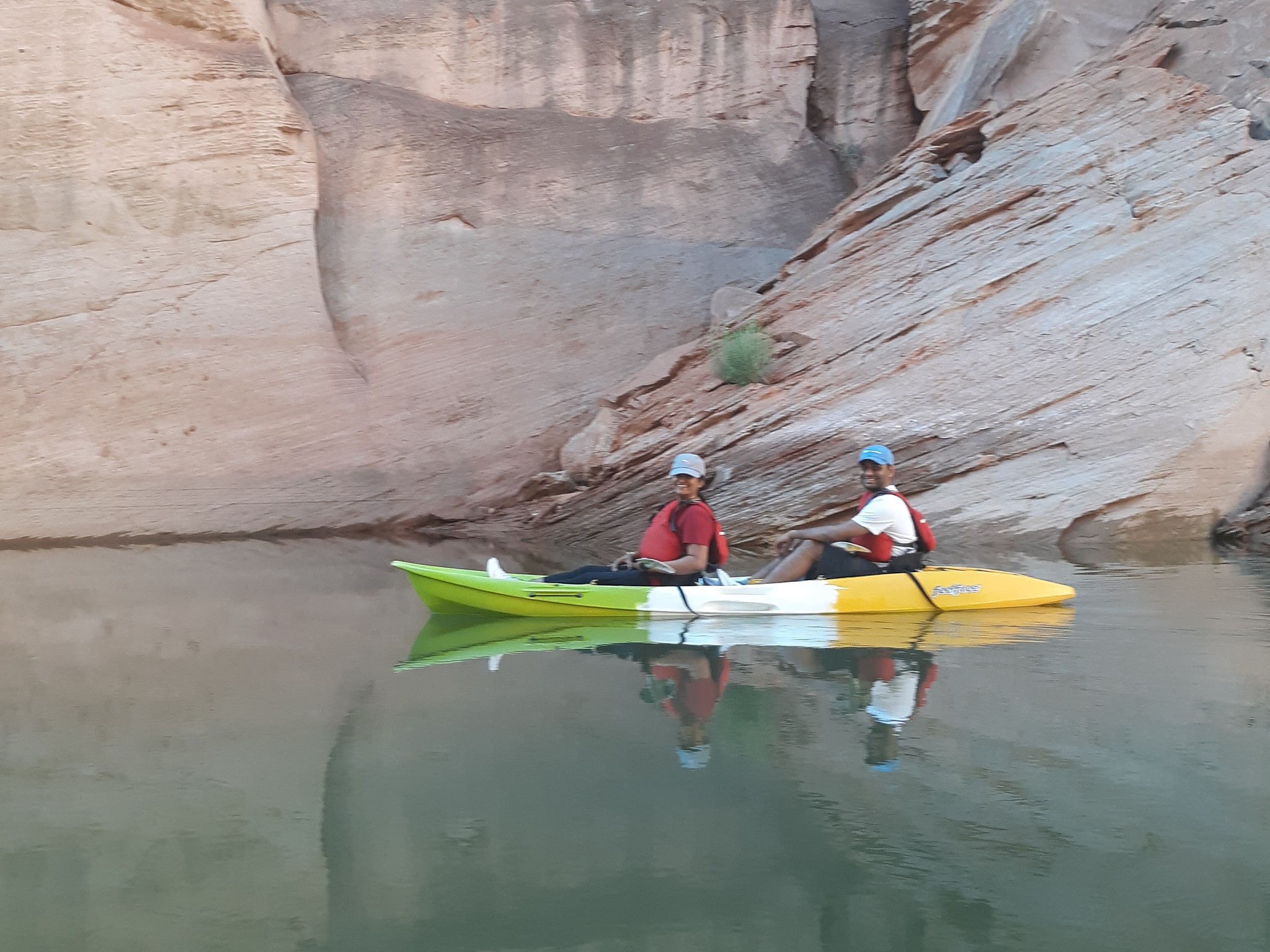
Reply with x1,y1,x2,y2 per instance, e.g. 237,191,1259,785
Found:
390,605,1078,675
388,556,1077,624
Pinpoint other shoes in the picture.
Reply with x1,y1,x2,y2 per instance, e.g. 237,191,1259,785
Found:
703,576,722,586
486,557,512,580
717,569,741,586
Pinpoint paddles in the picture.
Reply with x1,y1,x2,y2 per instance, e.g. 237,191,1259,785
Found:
606,558,677,574
830,542,872,554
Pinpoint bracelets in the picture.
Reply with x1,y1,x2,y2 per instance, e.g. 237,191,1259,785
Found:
626,552,636,554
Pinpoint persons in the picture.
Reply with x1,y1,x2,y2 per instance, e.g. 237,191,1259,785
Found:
486,453,714,586
719,644,921,774
702,444,919,585
488,644,720,771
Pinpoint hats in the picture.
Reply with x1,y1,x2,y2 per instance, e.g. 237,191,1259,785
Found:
668,453,706,478
857,444,895,465
